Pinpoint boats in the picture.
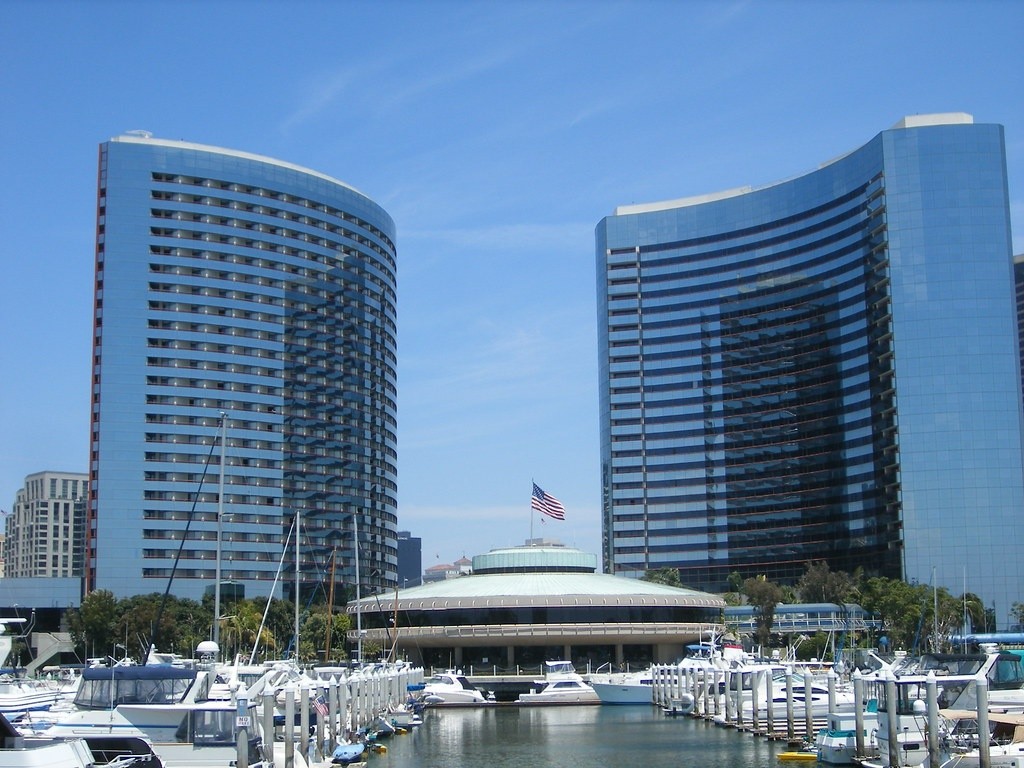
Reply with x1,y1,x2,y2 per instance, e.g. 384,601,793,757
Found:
515,661,600,702
591,666,705,706
421,674,485,704
678,645,1024,768
1,413,412,768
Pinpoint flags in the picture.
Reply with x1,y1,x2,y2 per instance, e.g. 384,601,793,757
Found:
532,482,566,520
312,694,328,716
542,519,547,526
